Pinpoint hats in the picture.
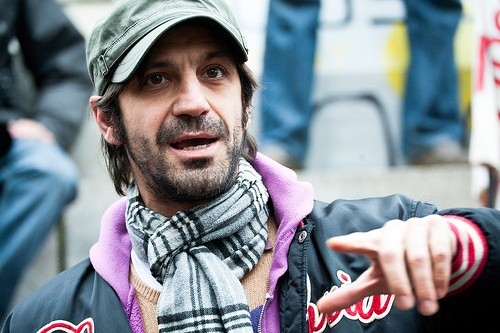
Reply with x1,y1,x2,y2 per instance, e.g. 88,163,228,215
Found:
85,0,248,98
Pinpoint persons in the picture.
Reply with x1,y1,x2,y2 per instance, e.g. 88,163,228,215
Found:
1,1,95,318
257,0,463,170
1,0,500,333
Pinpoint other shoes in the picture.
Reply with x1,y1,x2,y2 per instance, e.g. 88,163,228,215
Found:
411,146,468,165
261,147,301,170
